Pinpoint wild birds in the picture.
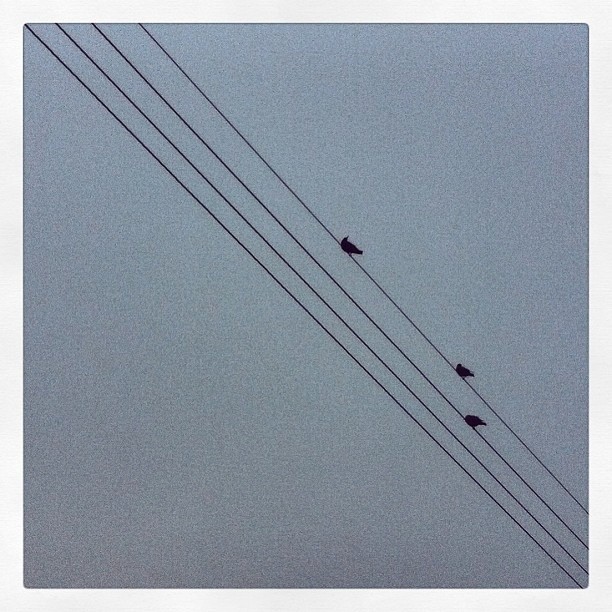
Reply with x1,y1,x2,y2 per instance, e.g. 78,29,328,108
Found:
340,235,363,257
456,364,475,381
464,415,487,430
